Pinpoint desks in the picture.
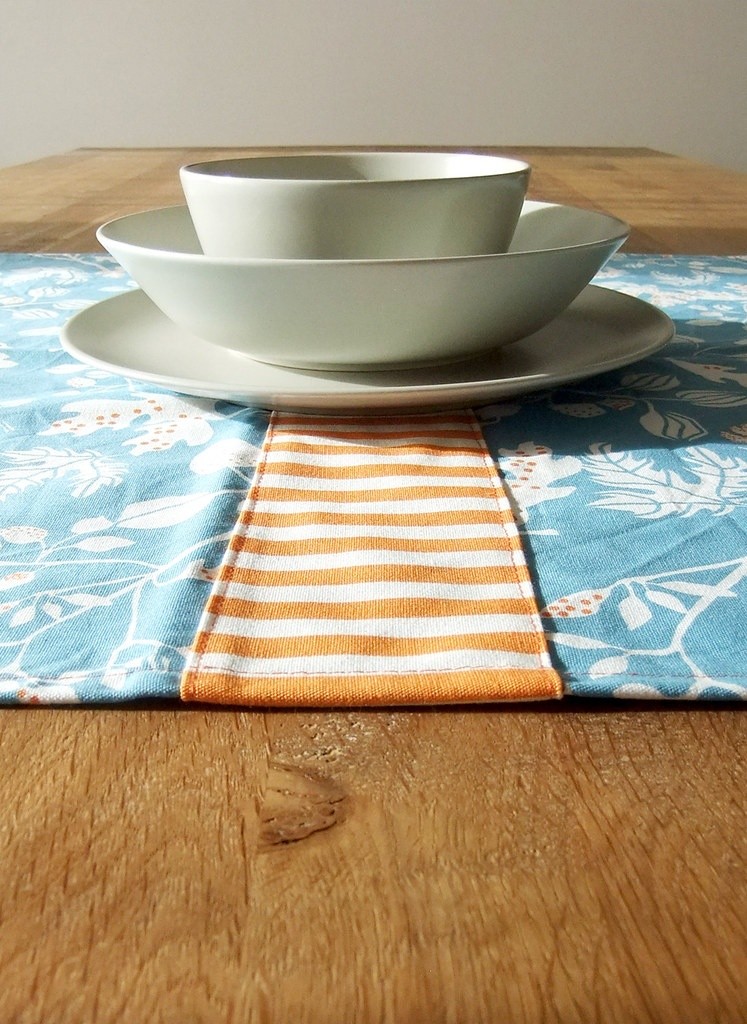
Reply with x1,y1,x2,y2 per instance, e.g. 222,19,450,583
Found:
0,146,747,1024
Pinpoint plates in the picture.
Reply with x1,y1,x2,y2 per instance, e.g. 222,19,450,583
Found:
59,288,677,418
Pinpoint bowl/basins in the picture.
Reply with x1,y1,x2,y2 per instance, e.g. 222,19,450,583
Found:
179,151,531,258
97,203,633,372
